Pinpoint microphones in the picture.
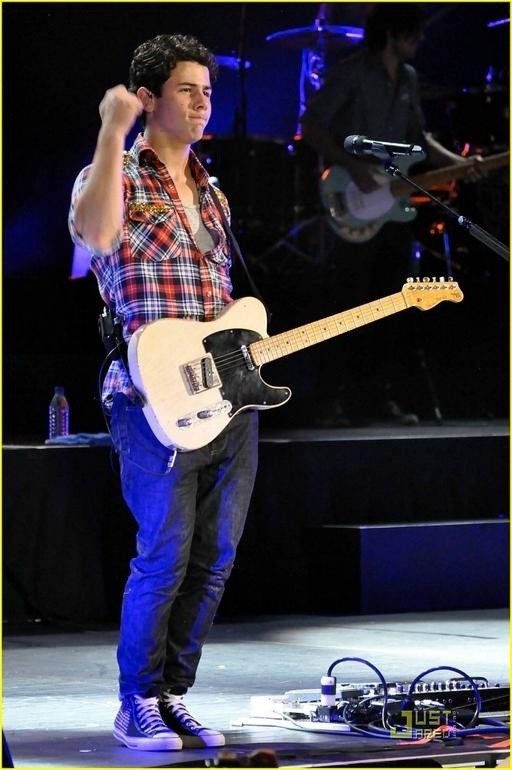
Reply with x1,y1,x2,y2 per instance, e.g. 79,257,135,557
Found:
344,134,423,165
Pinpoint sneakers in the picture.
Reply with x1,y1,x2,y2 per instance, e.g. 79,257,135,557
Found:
111,696,183,752
149,690,226,747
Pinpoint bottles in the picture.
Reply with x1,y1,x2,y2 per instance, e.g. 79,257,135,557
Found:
49,386,68,440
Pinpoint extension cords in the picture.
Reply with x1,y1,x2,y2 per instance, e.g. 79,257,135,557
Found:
310,701,477,731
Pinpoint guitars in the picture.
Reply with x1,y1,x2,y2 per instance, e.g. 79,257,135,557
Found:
126,275,466,452
319,142,508,243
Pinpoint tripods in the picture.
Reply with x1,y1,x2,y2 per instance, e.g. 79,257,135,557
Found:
254,42,345,268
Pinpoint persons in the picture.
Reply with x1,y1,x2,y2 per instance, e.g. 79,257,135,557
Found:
66,33,259,752
301,3,489,427
